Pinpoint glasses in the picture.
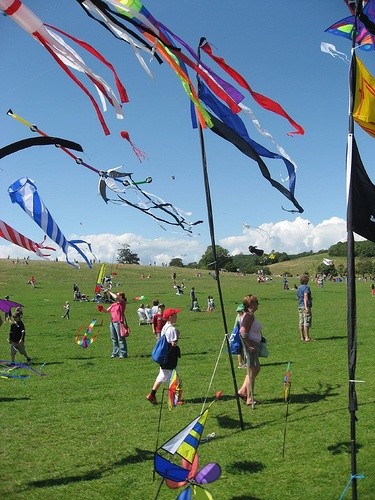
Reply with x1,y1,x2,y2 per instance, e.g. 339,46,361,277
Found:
251,300,258,304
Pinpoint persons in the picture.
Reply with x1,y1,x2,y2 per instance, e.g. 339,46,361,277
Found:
151,304,167,340
172,282,183,296
358,273,375,281
180,283,185,288
192,299,201,313
171,272,176,279
236,293,263,405
102,288,128,359
370,284,375,297
189,287,196,309
210,297,214,311
3,295,12,324
313,271,347,289
145,307,186,406
207,296,212,312
61,300,71,320
6,311,31,368
233,304,249,369
27,275,35,287
296,271,314,343
151,299,160,313
234,268,283,284
283,277,288,289
136,304,146,319
15,305,23,317
144,305,152,318
73,272,127,304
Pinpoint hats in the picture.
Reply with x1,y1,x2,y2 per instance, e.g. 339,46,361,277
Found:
159,303,165,308
236,304,245,312
163,308,181,321
13,312,20,317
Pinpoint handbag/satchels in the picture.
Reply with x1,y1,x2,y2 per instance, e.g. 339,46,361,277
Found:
152,326,175,365
226,321,243,354
259,329,269,358
120,312,131,337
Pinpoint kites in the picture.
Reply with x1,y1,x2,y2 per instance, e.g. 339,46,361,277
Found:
132,295,149,302
76,0,306,216
1,2,128,135
154,390,224,500
7,176,98,271
0,219,56,261
119,130,151,165
0,359,48,379
320,41,351,64
266,249,277,259
166,452,221,500
6,109,204,241
74,318,103,348
248,245,264,257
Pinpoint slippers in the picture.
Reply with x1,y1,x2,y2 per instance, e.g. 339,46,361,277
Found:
245,401,261,406
235,393,247,402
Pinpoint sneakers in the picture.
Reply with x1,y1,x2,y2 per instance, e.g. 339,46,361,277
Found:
301,338,304,341
111,355,116,358
177,400,184,405
242,363,248,366
5,363,16,367
238,365,248,369
305,337,317,342
119,354,124,359
27,359,32,366
146,393,158,405
